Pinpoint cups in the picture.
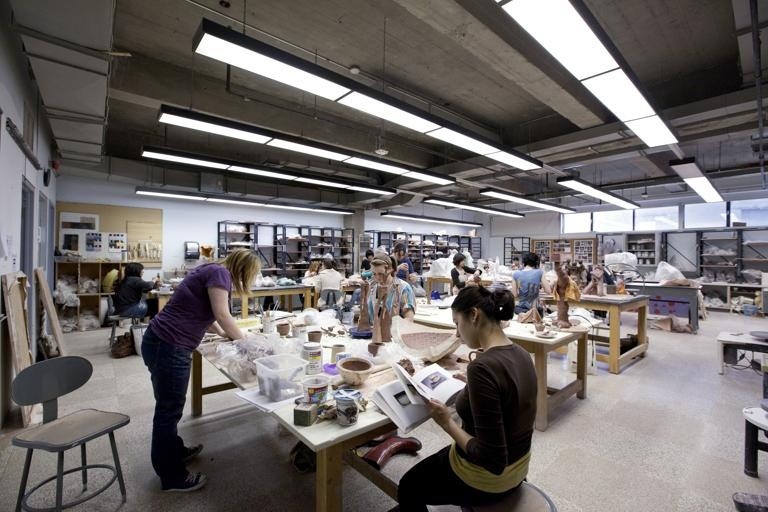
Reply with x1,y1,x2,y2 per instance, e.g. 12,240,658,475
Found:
301,376,329,404
262,318,275,334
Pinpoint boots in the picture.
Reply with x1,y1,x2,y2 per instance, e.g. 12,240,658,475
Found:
362,436,422,471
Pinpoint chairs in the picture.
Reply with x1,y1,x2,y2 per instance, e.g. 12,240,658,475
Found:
107,294,140,348
11,356,129,512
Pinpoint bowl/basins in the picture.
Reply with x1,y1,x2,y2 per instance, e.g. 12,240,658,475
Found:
277,324,290,336
337,357,374,386
308,331,322,342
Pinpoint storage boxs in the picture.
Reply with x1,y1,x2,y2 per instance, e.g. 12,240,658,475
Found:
252,354,308,402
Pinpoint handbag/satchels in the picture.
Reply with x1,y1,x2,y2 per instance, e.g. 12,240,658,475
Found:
109,332,134,359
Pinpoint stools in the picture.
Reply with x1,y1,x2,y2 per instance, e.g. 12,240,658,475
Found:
462,480,557,512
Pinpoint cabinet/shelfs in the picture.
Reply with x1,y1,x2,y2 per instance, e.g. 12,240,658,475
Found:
359,230,481,275
55,260,131,329
217,221,354,284
624,226,768,314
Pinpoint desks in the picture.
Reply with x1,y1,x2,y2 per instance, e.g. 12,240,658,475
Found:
314,283,369,308
608,283,699,333
403,312,589,432
514,294,648,374
427,277,492,302
717,330,768,375
232,286,312,319
192,312,501,512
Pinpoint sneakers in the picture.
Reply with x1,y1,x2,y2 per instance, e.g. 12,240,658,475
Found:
179,443,203,465
160,472,208,494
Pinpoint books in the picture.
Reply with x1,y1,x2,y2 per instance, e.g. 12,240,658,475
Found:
370,361,466,436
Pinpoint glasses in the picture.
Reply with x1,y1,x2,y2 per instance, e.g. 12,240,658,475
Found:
372,271,384,275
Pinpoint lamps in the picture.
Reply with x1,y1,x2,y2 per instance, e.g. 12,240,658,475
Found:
381,204,483,228
193,0,542,172
423,195,524,218
157,54,456,187
480,186,576,214
134,167,355,214
556,175,640,210
668,159,725,203
141,124,395,196
502,1,678,148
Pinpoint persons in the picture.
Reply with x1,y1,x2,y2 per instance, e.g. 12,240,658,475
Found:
389,242,408,282
367,253,416,326
393,240,414,281
450,252,482,290
112,261,155,320
511,257,523,270
512,252,552,310
387,285,539,512
140,247,262,493
360,250,375,271
304,259,320,278
314,257,342,307
